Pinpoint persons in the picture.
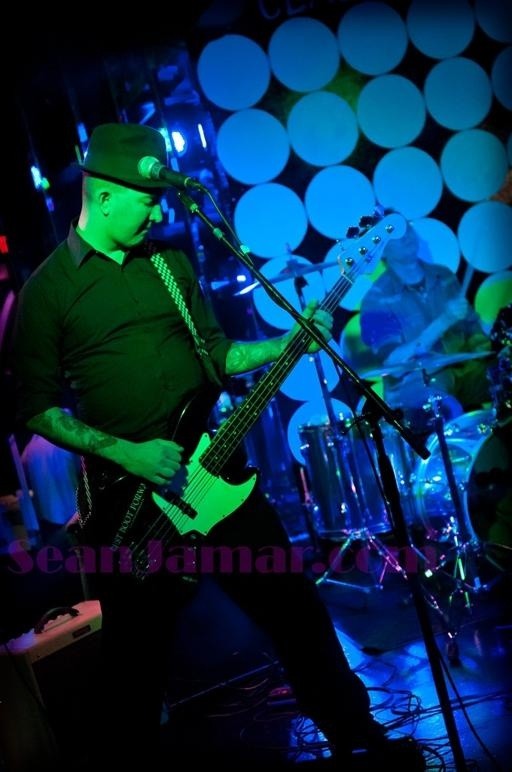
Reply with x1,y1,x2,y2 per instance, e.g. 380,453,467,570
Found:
19,407,81,543
0,118,390,766
362,220,495,408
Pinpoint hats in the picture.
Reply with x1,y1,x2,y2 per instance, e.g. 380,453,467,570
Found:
78,122,175,189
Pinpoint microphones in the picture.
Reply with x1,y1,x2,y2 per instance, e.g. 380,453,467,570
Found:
139,157,203,196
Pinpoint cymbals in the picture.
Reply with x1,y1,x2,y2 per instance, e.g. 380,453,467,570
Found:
232,262,340,296
361,350,494,378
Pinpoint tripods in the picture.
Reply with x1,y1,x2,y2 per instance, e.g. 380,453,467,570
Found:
404,388,512,625
292,274,409,597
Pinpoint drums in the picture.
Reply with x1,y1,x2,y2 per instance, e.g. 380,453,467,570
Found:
301,417,414,543
411,407,512,564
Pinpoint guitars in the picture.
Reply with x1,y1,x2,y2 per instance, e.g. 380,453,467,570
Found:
82,209,408,609
